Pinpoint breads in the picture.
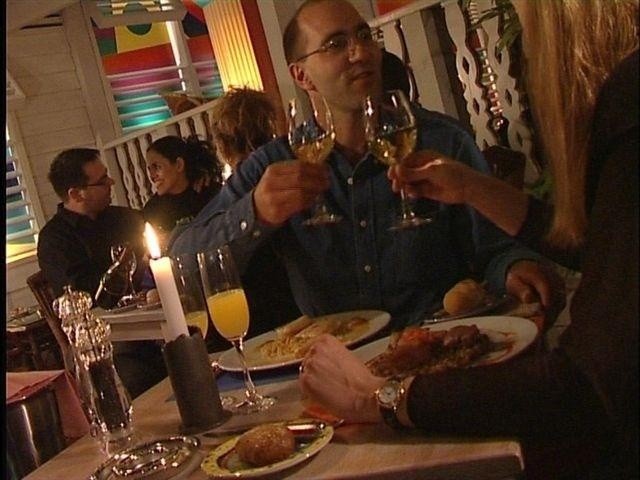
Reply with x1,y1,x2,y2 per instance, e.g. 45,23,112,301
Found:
146,289,160,302
442,278,484,315
236,424,295,464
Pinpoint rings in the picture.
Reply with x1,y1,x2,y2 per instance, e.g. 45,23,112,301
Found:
299,365,308,374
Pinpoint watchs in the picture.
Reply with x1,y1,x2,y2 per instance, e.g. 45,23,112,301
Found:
375,375,410,432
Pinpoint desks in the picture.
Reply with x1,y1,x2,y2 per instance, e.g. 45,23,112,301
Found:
6,370,64,479
7,304,64,371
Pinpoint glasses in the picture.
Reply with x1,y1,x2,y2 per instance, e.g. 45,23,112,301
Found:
295,26,378,62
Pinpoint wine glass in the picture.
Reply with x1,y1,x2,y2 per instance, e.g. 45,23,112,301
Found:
364,88,436,231
161,253,209,341
286,92,344,228
110,244,142,303
198,242,279,414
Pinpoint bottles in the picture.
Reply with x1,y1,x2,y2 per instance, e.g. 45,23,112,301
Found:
52,285,137,460
94,241,136,310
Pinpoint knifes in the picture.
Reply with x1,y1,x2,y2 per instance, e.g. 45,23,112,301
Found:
205,418,326,437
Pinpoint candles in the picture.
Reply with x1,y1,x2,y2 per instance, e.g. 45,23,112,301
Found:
142,222,193,341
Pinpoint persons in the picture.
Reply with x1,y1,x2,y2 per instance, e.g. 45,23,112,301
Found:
215,85,279,178
36,148,144,402
138,134,223,257
380,50,475,141
296,50,637,478
163,1,567,315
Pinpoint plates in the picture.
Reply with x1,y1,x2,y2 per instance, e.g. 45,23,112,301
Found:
200,422,334,477
348,314,539,378
218,308,392,373
88,432,203,480
423,292,494,323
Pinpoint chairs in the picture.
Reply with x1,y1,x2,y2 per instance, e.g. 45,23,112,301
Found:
27,270,81,386
